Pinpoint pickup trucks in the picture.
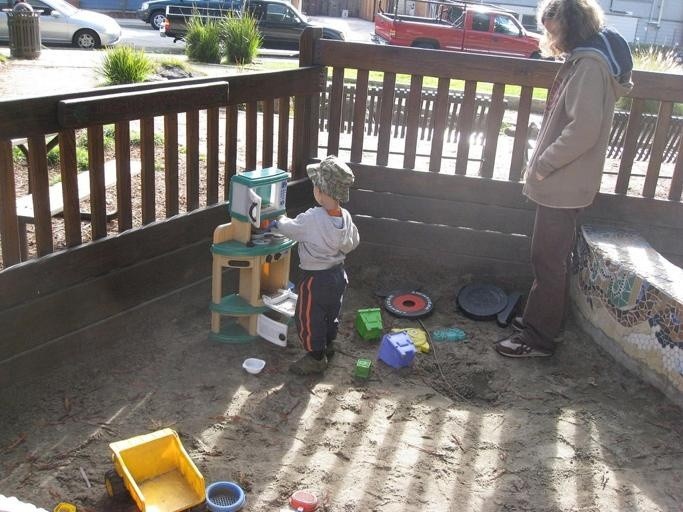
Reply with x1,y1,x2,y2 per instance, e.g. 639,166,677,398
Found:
164,0,344,52
375,1,546,60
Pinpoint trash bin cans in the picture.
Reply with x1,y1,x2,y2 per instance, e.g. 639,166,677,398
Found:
6,2,41,59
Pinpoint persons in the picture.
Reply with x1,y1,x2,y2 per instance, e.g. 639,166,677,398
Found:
12,0,34,16
260,155,362,377
495,0,635,359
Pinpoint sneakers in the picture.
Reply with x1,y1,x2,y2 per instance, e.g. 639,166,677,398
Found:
496,336,553,357
512,317,563,342
290,345,334,374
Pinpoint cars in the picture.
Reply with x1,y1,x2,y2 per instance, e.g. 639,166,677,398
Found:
0,0,123,49
136,0,294,30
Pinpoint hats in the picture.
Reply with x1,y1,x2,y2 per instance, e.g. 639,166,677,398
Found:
306,155,355,202
